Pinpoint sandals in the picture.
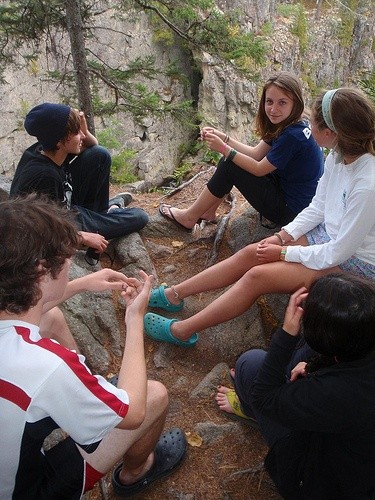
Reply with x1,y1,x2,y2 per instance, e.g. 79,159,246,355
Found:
109,376,119,388
113,428,186,496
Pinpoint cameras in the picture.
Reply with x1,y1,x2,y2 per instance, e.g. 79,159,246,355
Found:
85,246,101,261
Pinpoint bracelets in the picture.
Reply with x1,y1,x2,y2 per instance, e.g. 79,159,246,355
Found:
218,144,227,153
274,232,286,244
227,149,237,161
279,246,287,260
223,135,229,143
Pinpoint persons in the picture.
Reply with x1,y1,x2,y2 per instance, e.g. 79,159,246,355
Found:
143,88,374,347
159,73,325,232
0,191,188,500
214,273,375,500
9,102,148,254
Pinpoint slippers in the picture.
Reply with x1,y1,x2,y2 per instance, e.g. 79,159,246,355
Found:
219,390,261,424
198,218,218,225
148,285,183,312
159,204,192,232
144,312,199,346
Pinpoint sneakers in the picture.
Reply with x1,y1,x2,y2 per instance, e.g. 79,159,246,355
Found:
109,193,132,209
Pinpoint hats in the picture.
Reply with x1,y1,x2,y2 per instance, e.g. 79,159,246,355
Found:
24,103,71,149
